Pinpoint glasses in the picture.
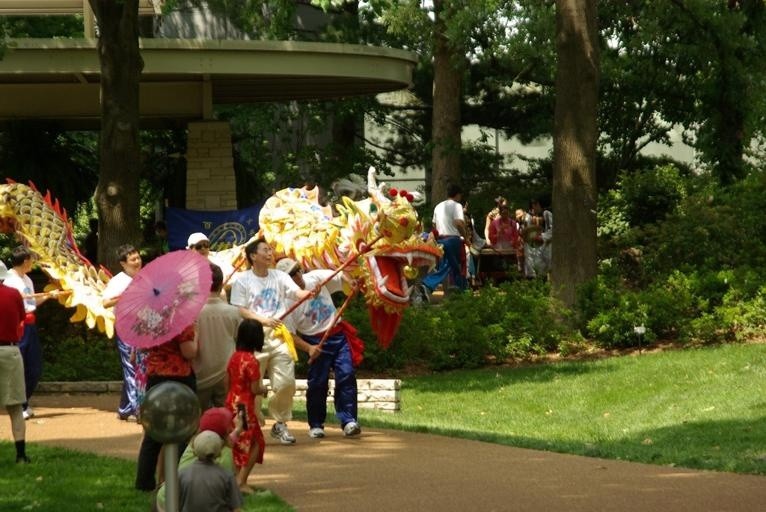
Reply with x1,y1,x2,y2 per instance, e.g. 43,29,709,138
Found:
190,242,210,250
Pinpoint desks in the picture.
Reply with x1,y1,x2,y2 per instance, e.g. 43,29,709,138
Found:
475,248,523,289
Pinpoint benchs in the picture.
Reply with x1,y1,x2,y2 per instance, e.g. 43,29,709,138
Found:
285,3,329,42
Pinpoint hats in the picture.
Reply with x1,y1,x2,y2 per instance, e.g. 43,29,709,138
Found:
0,259,8,281
185,232,210,250
200,406,234,438
276,258,297,277
193,430,222,459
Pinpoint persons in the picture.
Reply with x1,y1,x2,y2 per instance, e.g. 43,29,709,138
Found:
223,318,268,494
419,181,553,305
231,239,320,445
100,243,153,422
186,229,250,411
2,246,63,465
152,404,244,511
130,327,196,491
276,257,359,438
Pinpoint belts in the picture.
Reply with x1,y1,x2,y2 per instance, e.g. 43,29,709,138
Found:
0,340,19,348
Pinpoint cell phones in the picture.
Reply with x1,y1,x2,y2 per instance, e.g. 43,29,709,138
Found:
238,403,248,429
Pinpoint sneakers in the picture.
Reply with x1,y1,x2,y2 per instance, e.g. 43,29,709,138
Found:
342,422,361,437
419,283,432,303
307,426,325,438
23,405,34,420
120,411,137,423
15,455,32,465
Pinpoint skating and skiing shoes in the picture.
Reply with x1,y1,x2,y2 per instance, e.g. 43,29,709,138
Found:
270,421,297,445
